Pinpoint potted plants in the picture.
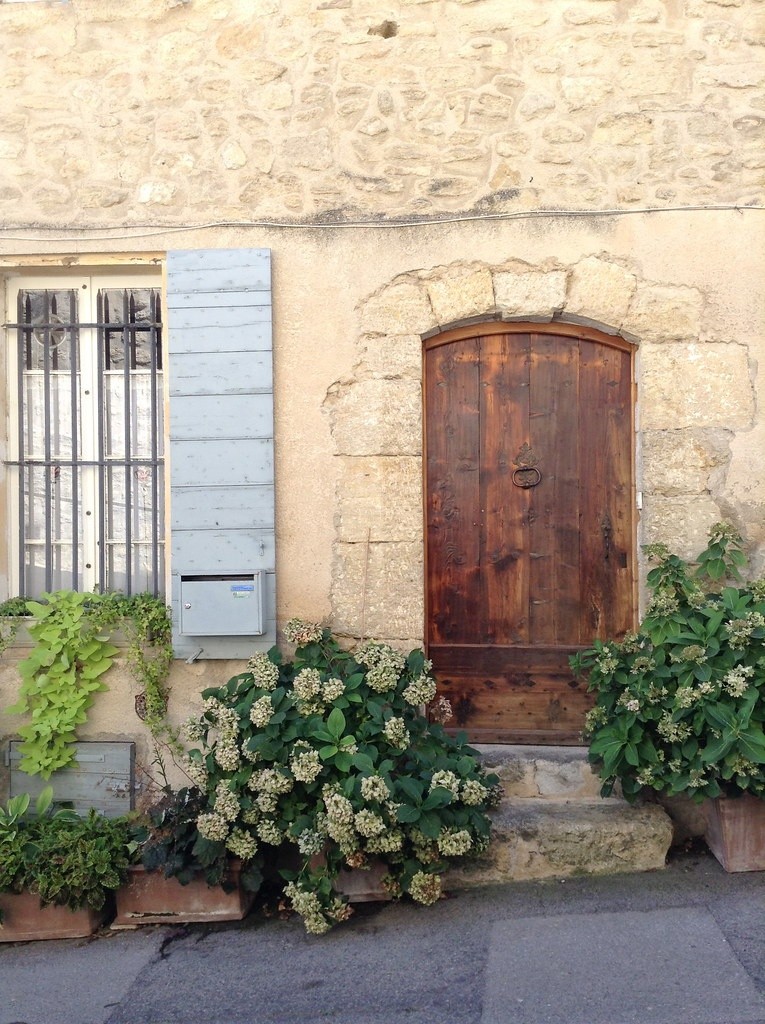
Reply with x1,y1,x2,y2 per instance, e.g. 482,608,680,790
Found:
182,620,507,936
1,787,144,942
109,786,259,931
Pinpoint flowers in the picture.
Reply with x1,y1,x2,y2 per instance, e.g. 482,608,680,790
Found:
570,520,764,804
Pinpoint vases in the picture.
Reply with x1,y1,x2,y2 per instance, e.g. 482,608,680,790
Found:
309,846,393,903
700,795,765,873
